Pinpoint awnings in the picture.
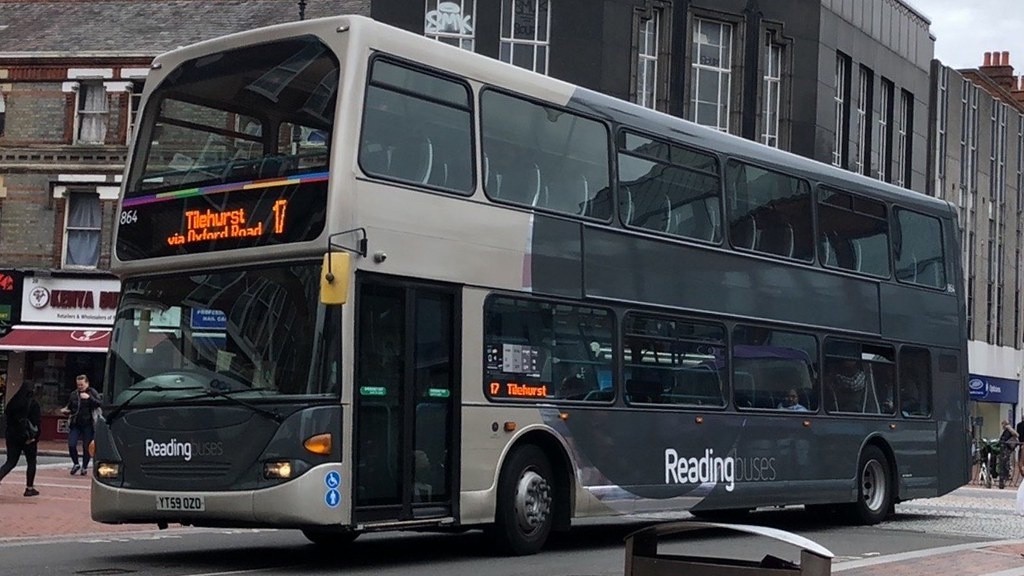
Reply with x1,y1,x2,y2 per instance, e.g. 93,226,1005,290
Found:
0,325,112,352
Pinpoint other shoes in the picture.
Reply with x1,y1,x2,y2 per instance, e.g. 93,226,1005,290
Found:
81,467,87,475
70,464,80,474
24,488,39,496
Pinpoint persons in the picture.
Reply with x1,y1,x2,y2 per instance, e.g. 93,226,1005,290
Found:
0,379,41,497
826,350,867,412
775,384,808,412
966,414,1024,490
60,374,104,477
877,362,920,418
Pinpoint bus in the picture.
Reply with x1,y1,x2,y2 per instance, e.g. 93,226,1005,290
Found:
90,14,975,554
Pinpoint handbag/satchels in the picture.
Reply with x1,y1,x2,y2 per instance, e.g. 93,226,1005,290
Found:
1006,436,1020,449
19,418,38,444
67,414,76,429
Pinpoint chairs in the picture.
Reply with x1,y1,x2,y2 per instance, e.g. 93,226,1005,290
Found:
208,135,947,288
544,358,928,417
